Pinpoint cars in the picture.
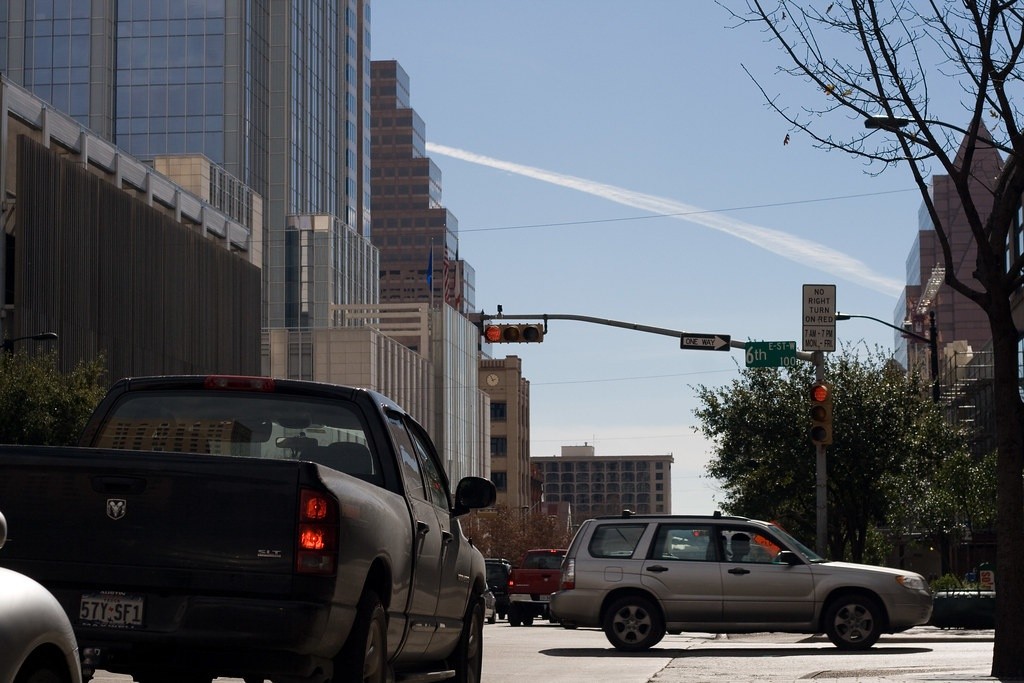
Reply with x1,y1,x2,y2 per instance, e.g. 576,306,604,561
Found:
605,535,700,559
482,581,499,624
1,513,82,681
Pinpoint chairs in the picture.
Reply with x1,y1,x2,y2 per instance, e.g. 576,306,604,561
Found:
316,442,372,478
706,534,728,565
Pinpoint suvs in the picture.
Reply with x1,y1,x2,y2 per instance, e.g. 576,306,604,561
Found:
482,557,513,624
548,510,935,652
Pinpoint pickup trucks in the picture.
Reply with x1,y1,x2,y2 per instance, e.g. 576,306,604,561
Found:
506,548,570,626
1,373,498,682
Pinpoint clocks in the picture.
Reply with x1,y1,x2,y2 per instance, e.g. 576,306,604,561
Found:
486,373,499,387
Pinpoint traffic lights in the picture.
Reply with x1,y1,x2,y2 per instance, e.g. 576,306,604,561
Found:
692,529,710,537
809,382,833,446
485,323,544,344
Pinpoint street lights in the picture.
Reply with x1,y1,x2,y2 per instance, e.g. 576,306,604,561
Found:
834,311,941,405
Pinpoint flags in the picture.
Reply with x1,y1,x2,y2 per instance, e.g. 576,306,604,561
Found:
427,240,462,306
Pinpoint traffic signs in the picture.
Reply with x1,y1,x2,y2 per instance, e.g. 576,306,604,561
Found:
680,332,731,352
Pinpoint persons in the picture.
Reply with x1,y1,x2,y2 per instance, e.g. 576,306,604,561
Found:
730,533,752,562
